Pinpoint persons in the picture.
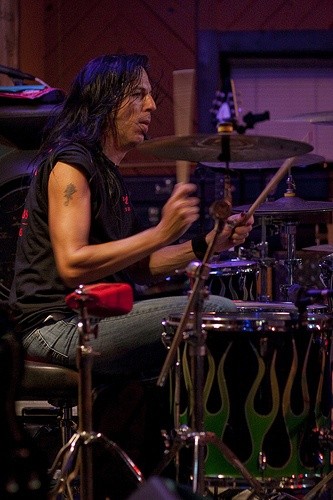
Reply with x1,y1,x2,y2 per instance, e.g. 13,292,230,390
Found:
8,52,239,370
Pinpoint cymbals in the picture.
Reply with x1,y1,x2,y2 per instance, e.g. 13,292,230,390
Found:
304,245,332,252
136,133,314,161
231,197,333,216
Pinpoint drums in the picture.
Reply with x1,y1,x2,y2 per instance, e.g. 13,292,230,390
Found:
163,302,333,489
203,261,257,301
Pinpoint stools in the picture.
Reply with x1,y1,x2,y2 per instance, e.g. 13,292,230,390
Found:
20,360,80,500
